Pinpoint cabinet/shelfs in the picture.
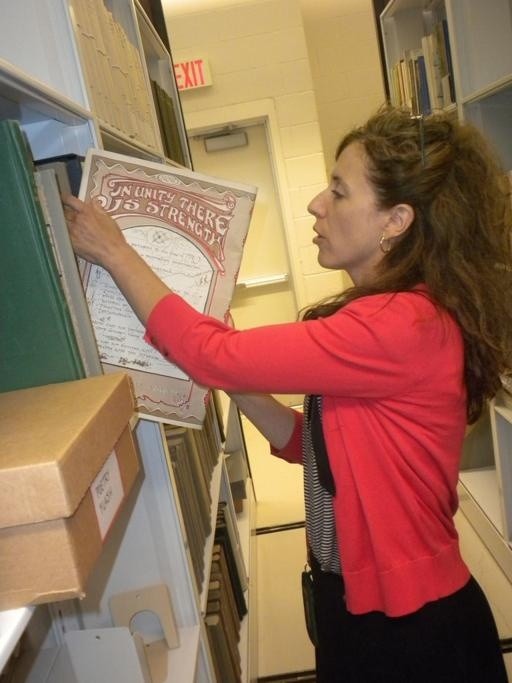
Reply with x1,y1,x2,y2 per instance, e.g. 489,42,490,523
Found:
0,0,259,683
373,0,512,580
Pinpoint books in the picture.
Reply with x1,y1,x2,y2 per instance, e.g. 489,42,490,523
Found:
151,77,187,167
205,500,249,678
166,393,238,598
1,117,115,380
389,21,456,119
70,0,159,150
77,147,257,432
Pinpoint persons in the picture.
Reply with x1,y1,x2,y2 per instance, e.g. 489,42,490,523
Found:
59,104,512,678
